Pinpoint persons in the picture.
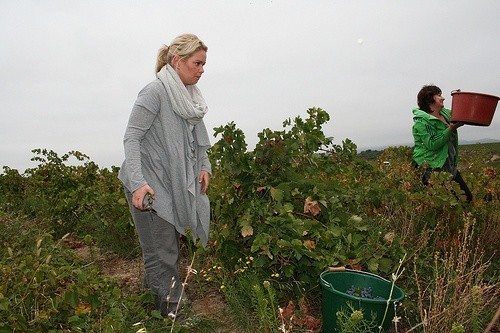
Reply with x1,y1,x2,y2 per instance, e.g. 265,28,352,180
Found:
117,33,211,324
412,84,473,209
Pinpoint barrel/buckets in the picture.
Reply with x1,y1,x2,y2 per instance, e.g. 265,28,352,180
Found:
319,266,405,333
450,89,500,126
